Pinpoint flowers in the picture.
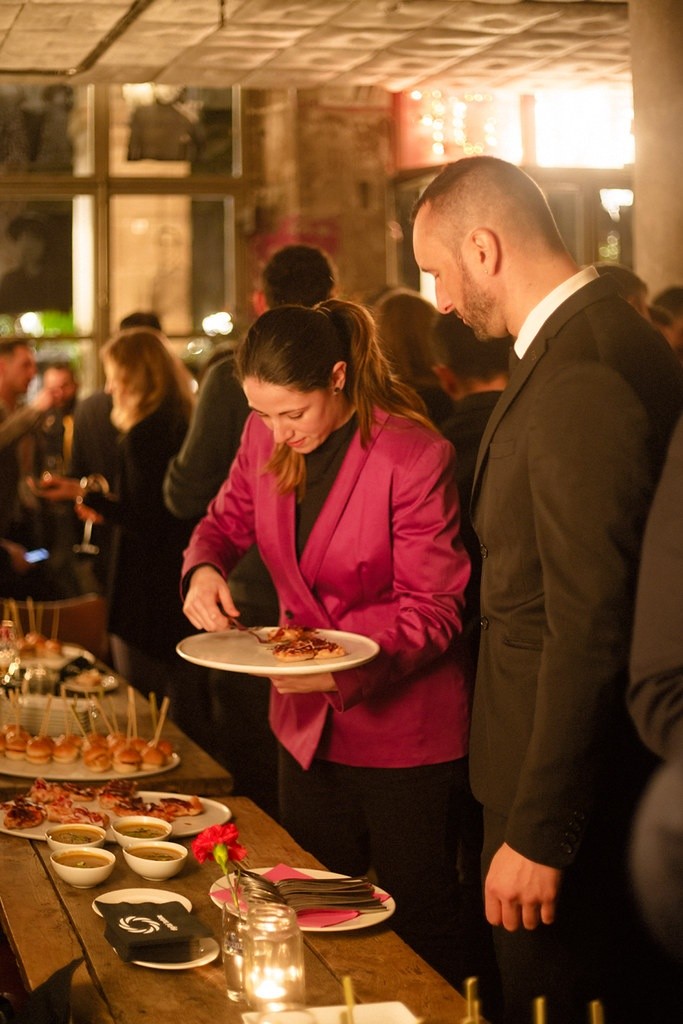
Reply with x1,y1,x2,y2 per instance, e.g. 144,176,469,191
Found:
194,821,247,919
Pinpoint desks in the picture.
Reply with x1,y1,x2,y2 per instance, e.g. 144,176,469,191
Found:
1,794,483,1024
0,666,235,802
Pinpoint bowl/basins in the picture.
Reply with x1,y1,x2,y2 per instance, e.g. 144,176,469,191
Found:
44,823,107,852
122,840,188,882
50,846,116,889
111,816,172,848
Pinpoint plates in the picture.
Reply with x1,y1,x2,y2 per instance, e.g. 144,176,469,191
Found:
92,888,193,918
0,751,181,782
1,791,231,840
176,626,381,674
113,938,220,969
209,867,396,932
241,1000,418,1024
18,695,96,737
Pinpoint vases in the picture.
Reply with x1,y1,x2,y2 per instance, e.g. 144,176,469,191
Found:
223,897,265,1004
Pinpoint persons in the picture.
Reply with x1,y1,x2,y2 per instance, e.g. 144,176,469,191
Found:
0,312,214,751
592,263,683,1024
166,246,512,965
415,156,670,1024
177,300,472,977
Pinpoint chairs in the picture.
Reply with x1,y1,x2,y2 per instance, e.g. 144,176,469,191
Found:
17,594,108,663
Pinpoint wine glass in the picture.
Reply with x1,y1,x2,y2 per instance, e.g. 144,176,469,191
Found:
74,476,108,554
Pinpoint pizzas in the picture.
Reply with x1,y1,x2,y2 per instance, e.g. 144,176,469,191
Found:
270,621,346,662
0,777,202,829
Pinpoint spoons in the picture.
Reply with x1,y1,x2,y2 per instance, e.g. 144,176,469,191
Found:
233,869,388,918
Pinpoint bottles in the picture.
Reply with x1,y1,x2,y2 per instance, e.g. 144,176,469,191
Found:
241,903,305,1014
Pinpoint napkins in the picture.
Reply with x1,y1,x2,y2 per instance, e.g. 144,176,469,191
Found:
202,863,391,928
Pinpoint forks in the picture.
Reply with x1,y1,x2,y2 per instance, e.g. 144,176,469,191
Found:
224,616,283,644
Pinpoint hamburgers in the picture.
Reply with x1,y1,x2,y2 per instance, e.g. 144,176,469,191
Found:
0,724,173,774
